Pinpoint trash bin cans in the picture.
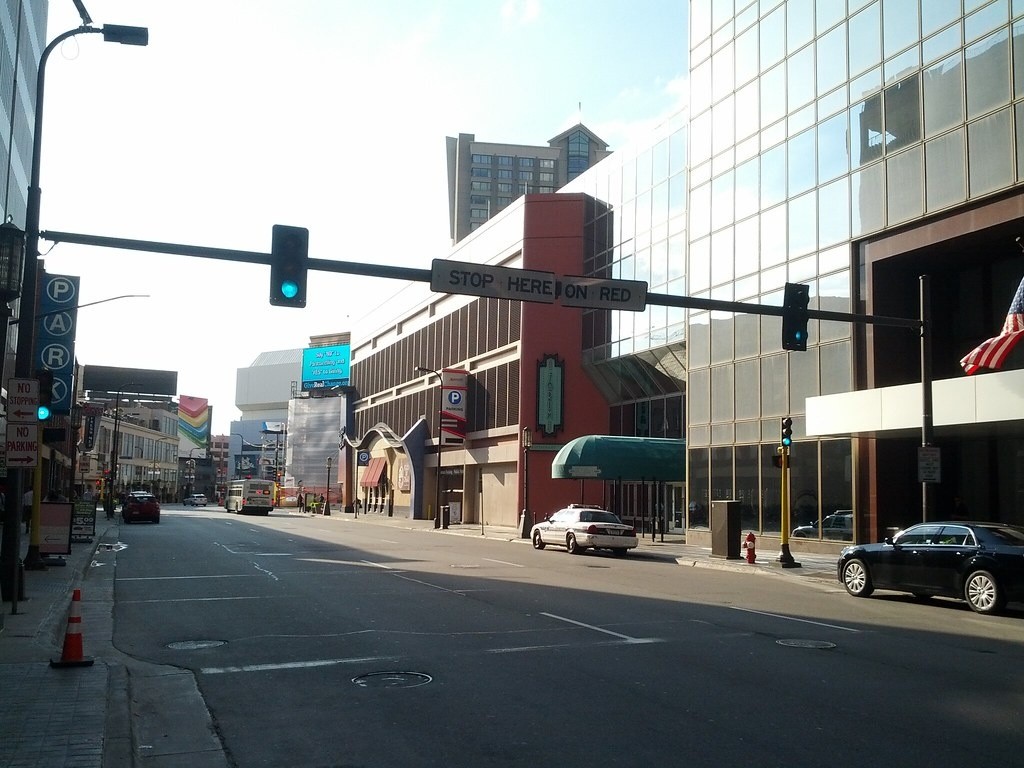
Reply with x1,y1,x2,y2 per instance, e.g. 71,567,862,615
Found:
311,503,318,513
440,506,450,529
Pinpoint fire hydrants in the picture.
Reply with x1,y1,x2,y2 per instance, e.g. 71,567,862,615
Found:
741,532,757,564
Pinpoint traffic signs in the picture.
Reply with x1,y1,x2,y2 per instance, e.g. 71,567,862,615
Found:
5,378,39,469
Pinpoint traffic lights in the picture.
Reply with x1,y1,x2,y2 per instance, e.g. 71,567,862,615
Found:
782,282,810,352
104,468,111,476
96,479,101,490
269,225,309,308
780,416,793,447
37,369,53,421
277,473,280,481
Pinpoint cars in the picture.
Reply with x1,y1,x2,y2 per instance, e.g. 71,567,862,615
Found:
530,504,639,556
836,521,1024,615
121,491,161,524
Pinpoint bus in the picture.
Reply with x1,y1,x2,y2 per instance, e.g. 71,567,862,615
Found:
223,474,274,516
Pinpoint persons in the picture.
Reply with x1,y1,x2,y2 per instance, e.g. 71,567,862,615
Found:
23,488,33,535
43,488,68,502
297,494,304,512
319,493,324,503
314,493,318,503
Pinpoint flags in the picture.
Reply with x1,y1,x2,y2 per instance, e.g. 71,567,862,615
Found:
958,276,1024,377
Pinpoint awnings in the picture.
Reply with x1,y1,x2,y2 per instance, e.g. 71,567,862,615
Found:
550,435,686,544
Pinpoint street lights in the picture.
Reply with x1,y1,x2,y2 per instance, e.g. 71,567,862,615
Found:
518,427,535,538
0,23,149,603
68,404,84,502
232,432,242,479
188,448,200,496
152,437,167,494
106,384,143,519
414,367,443,529
323,457,332,516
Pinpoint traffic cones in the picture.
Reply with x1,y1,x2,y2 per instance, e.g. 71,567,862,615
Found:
50,589,94,669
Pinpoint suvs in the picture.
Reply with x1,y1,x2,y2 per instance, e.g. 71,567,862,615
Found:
183,493,207,507
791,510,853,541
